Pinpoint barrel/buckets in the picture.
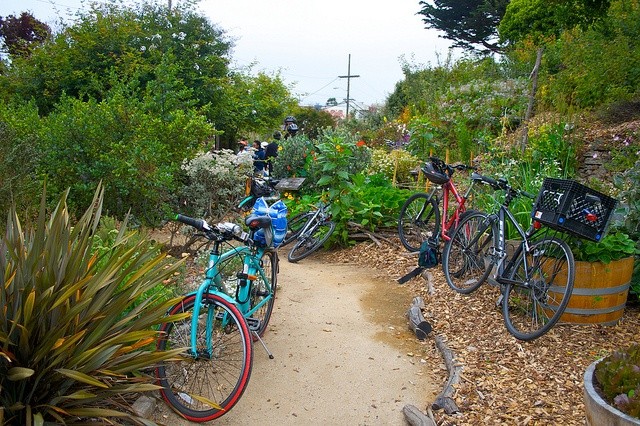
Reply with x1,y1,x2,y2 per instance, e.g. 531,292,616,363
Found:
522,252,634,326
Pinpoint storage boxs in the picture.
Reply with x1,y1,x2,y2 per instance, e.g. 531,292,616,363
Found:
531,177,618,244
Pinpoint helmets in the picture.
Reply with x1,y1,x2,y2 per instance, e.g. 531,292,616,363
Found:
421,162,450,184
285,116,297,123
287,124,299,130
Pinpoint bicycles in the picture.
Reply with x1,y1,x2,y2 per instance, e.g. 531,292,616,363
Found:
280,193,336,262
237,173,279,209
443,175,617,341
154,214,280,421
399,159,489,277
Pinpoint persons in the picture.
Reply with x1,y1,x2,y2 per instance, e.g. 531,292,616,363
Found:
287,124,299,138
261,142,269,176
250,140,265,175
241,136,251,152
236,141,247,156
264,131,282,176
284,116,297,140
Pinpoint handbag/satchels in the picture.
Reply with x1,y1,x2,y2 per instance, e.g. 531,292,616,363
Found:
252,196,287,251
397,235,440,284
